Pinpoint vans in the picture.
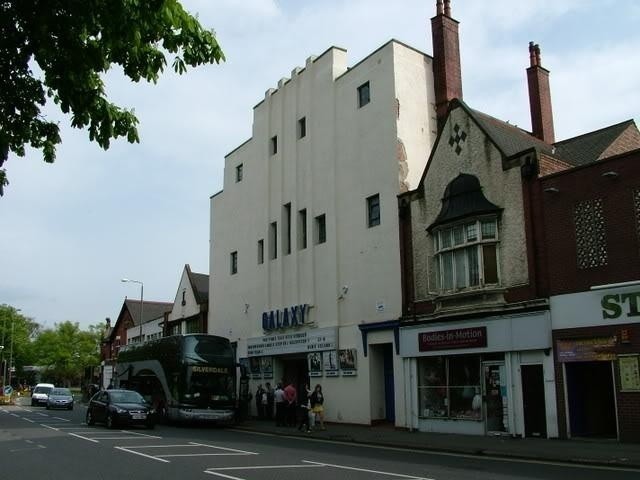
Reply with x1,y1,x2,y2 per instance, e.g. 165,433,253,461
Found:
31,384,55,406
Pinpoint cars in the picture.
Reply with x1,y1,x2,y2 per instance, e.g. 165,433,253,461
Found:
48,388,73,409
85,389,157,427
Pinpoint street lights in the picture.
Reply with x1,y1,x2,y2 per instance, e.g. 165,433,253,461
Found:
120,278,143,340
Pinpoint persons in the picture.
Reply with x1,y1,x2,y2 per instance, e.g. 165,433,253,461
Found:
255,379,326,433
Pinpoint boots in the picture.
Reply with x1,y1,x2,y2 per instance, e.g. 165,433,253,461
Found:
297,425,326,433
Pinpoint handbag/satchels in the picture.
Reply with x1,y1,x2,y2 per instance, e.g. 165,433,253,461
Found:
309,412,315,427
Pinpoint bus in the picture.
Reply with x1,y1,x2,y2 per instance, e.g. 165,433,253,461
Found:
117,334,248,421
